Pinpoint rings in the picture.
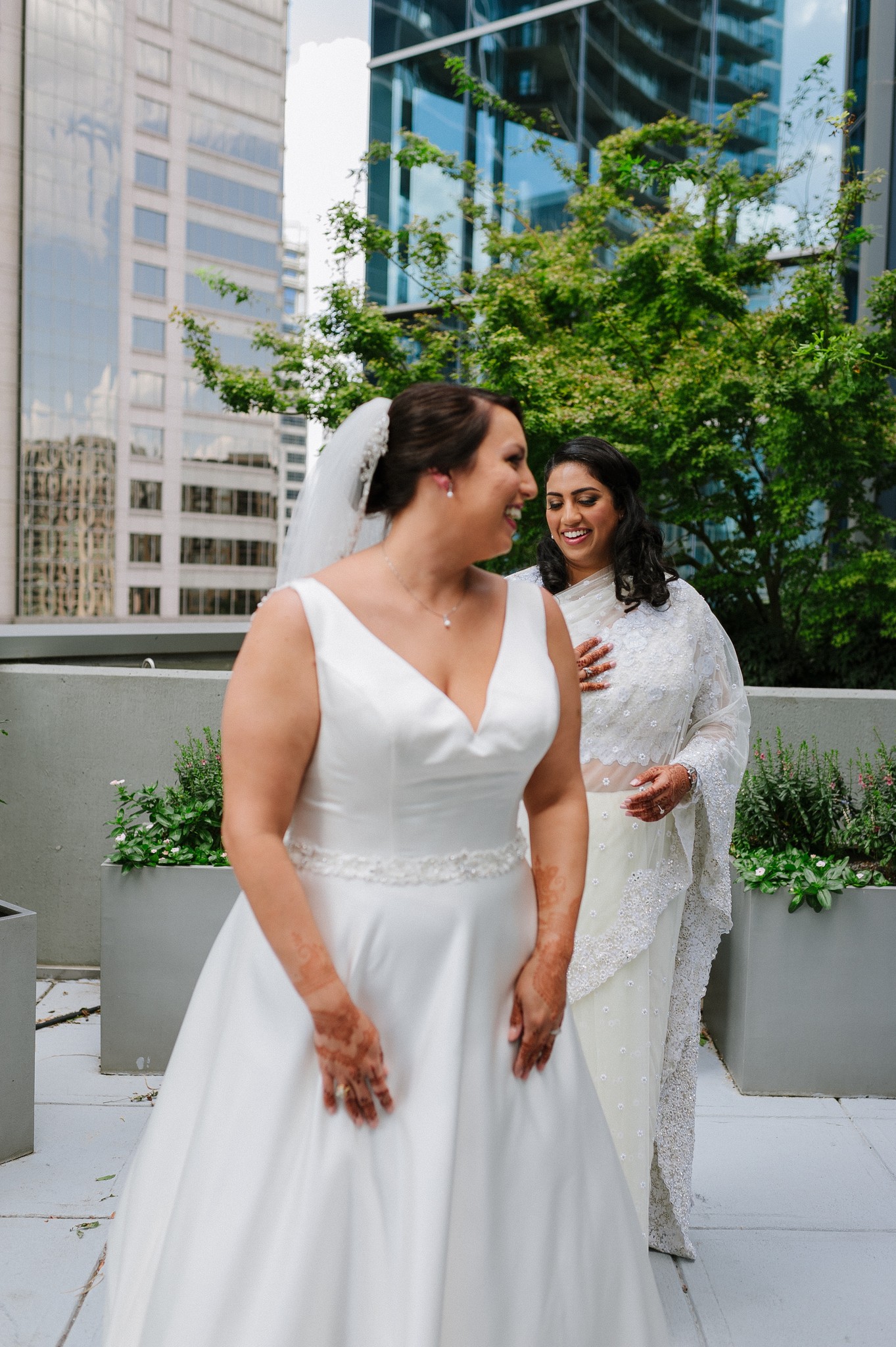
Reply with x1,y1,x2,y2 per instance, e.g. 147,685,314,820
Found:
335,1083,351,1100
549,1026,561,1035
657,805,665,814
583,667,593,679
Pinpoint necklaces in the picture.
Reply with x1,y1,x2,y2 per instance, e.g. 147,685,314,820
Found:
381,536,468,627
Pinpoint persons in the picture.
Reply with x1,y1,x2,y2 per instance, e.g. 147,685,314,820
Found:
495,439,751,1254
112,384,679,1347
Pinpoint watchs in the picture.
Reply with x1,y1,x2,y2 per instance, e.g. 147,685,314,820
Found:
678,762,698,794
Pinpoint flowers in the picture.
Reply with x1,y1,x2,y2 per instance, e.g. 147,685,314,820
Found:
101,725,232,877
728,725,896,915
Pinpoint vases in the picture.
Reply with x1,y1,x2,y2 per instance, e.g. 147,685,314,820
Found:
97,846,256,1080
706,853,896,1101
0,898,39,1168
